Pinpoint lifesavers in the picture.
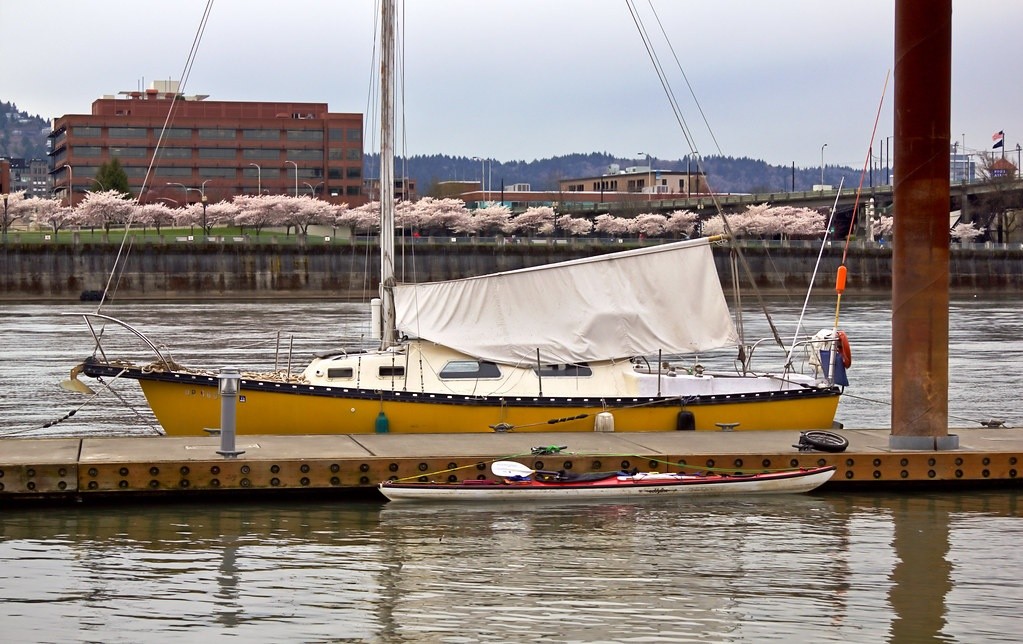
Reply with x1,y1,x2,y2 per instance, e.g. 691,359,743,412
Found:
804,429,850,453
837,331,851,369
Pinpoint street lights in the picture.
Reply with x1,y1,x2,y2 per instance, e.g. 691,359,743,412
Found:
821,143,827,191
284,161,298,198
186,179,213,207
638,152,651,201
302,181,324,199
473,157,486,209
686,151,699,198
249,162,261,195
63,164,72,213
166,182,187,209
85,176,104,193
202,196,208,235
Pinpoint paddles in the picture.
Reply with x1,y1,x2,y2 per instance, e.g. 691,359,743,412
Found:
490,460,560,476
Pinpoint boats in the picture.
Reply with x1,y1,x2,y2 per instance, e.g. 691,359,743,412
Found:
376,464,837,502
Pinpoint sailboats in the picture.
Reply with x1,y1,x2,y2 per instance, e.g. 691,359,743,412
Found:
60,0,889,431
